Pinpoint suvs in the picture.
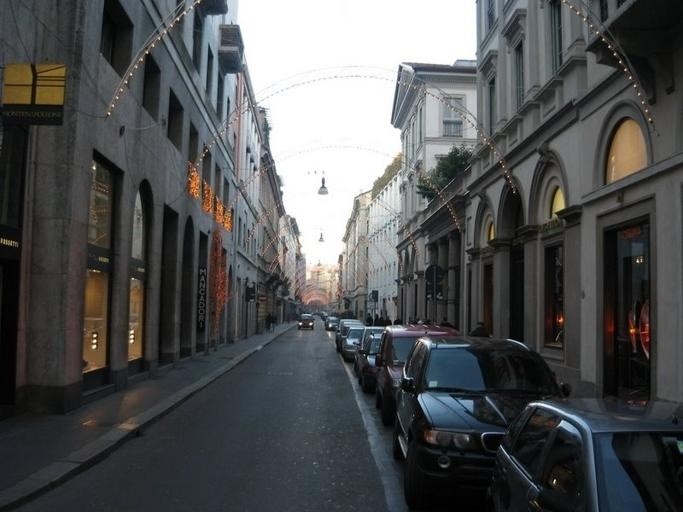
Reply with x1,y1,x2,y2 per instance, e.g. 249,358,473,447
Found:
396,334,570,511
376,324,466,425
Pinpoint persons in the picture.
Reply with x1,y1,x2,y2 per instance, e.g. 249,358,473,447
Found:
364,313,489,337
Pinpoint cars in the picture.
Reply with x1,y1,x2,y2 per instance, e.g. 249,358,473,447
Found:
321,311,339,331
299,314,316,330
335,319,383,394
492,395,683,512
